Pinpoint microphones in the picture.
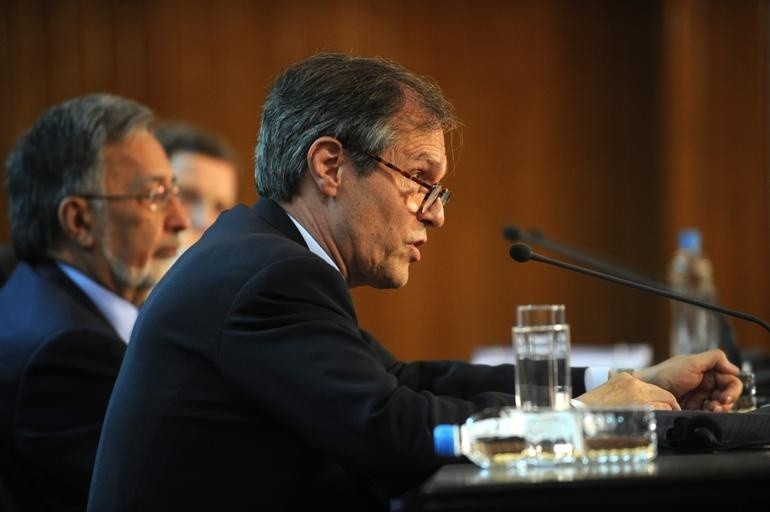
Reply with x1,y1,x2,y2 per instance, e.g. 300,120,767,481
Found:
508,242,770,415
524,228,770,369
502,224,770,397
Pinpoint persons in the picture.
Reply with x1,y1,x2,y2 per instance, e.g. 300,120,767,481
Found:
87,52,743,512
153,120,239,266
1,92,190,511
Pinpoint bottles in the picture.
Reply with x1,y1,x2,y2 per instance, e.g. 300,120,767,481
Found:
669,230,719,356
432,403,659,470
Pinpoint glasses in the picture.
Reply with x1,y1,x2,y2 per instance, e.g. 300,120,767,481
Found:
54,184,179,214
362,149,453,213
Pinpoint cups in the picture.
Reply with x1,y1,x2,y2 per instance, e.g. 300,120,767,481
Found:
510,302,573,409
